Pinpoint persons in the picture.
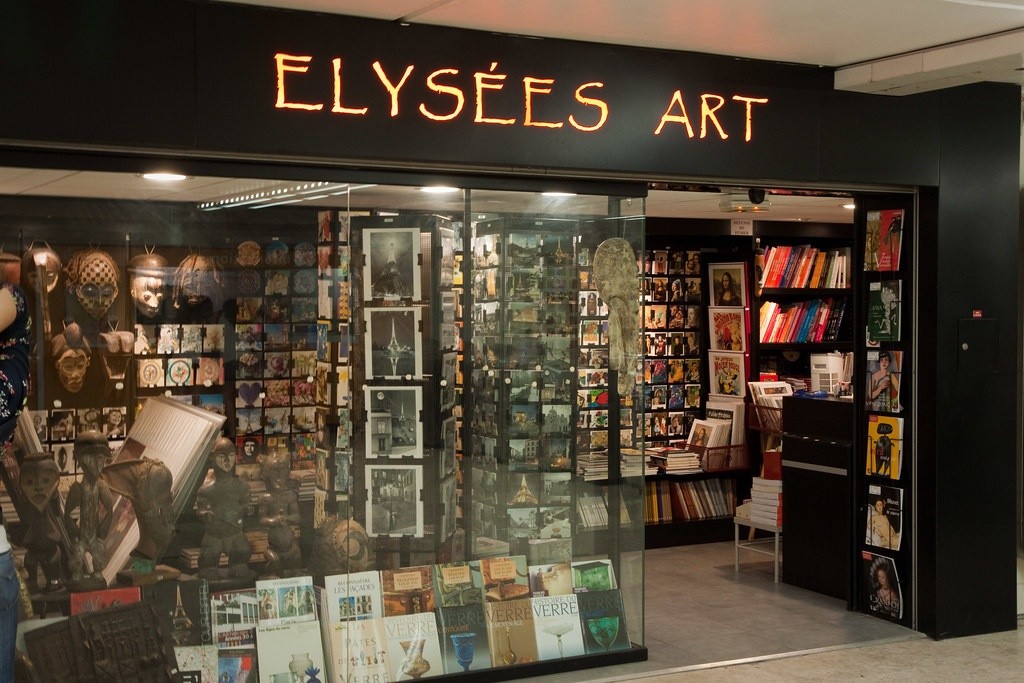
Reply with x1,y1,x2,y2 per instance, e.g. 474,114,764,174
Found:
196,437,253,578
19,451,64,594
687,304,700,328
869,498,897,547
656,279,666,300
875,568,899,613
719,272,738,306
0,285,31,682
691,428,705,455
258,459,303,576
63,430,113,572
670,414,681,434
869,352,899,413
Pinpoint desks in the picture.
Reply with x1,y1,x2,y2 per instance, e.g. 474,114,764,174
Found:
734,517,782,583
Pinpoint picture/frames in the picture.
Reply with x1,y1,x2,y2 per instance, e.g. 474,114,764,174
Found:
365,386,424,459
708,305,746,353
707,351,747,396
364,307,423,380
685,419,717,466
362,228,421,301
365,464,424,537
708,262,746,307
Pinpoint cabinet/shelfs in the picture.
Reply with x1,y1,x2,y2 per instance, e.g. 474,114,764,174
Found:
1,194,917,683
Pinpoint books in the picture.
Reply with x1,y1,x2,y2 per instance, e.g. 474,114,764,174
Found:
134,211,701,683
739,210,903,620
